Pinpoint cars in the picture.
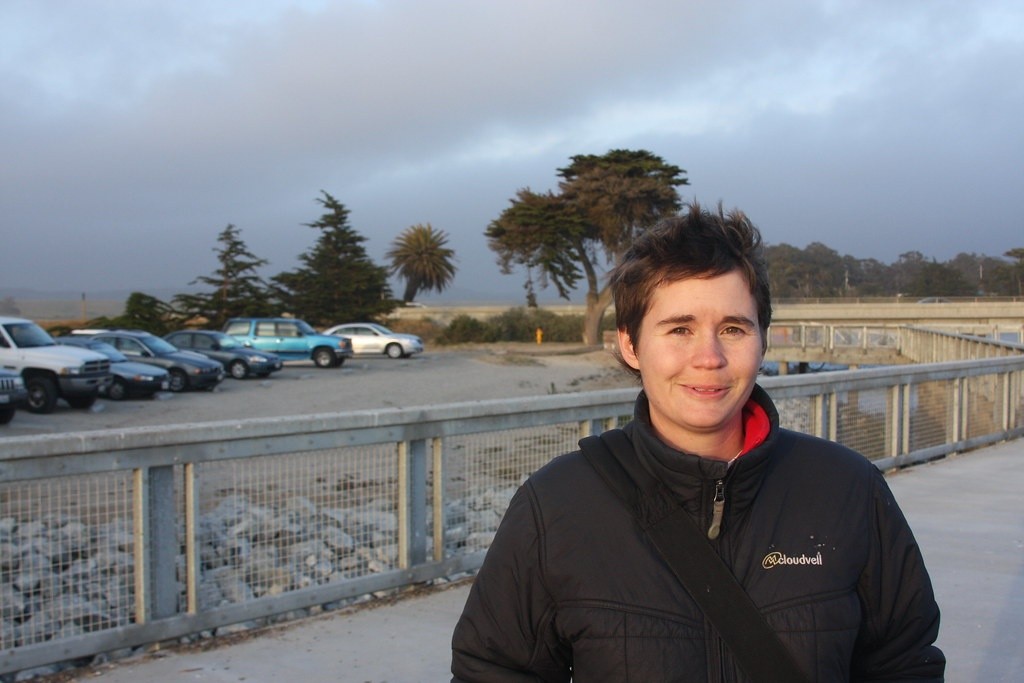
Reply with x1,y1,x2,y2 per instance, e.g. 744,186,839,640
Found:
71,328,226,393
323,322,424,359
55,336,171,401
916,296,952,303
163,330,282,380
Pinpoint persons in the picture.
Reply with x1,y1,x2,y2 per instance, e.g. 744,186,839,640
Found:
450,197,948,683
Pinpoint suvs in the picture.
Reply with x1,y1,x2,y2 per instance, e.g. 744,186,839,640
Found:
0,316,114,425
220,318,354,369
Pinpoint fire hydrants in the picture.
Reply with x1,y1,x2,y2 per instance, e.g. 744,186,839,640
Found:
535,328,543,344
